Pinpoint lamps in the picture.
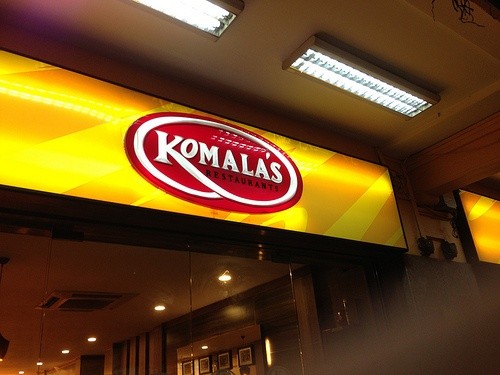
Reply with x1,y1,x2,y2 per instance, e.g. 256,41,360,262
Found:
283,36,440,122
131,0,242,42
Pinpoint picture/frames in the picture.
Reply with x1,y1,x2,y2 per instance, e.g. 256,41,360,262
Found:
180,358,195,375
237,344,256,367
216,350,233,371
198,354,213,374
